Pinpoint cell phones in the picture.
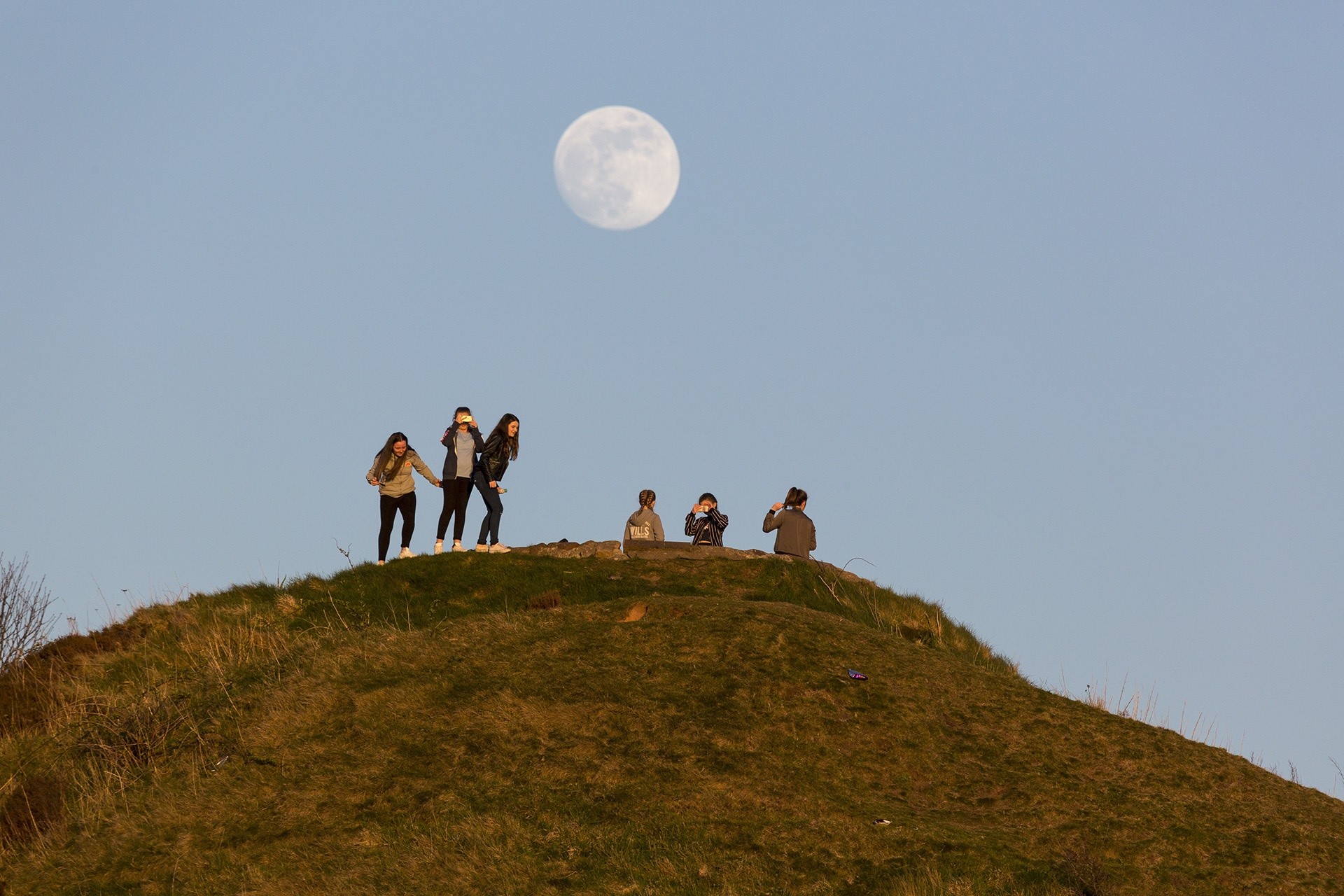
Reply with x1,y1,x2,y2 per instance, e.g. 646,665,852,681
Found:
461,416,472,422
504,489,507,492
698,505,709,512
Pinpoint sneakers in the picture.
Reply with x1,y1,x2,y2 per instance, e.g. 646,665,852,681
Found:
434,543,443,555
452,543,467,552
475,544,489,553
377,562,385,565
489,542,511,554
399,547,417,559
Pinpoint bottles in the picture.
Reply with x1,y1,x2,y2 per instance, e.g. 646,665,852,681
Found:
498,488,507,494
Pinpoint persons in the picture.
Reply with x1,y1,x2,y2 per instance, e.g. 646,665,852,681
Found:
762,487,818,557
434,407,485,556
366,432,443,565
471,414,520,554
623,490,663,548
685,492,730,547
